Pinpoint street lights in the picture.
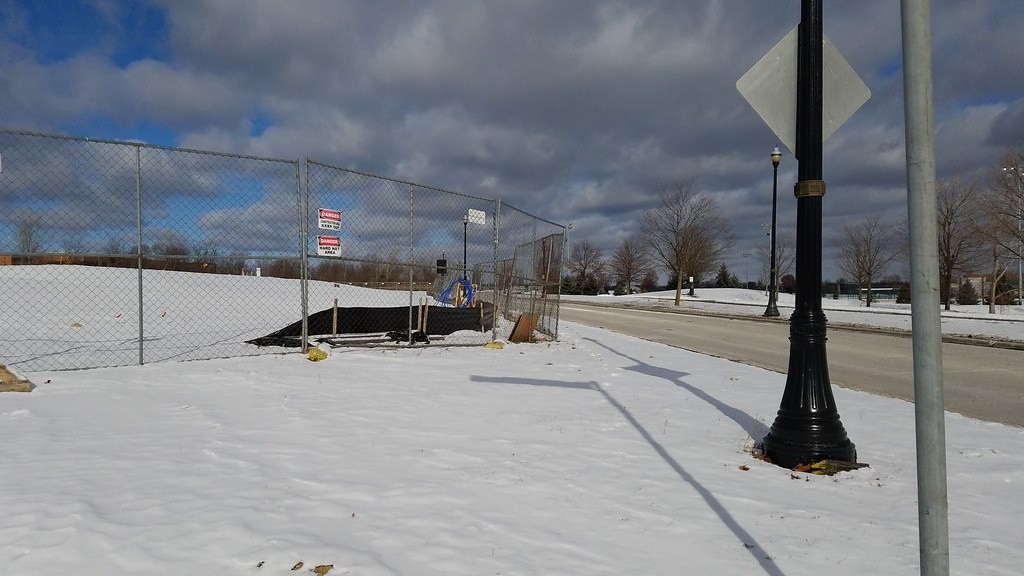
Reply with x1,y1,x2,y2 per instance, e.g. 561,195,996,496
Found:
763,145,780,317
463,214,468,297
